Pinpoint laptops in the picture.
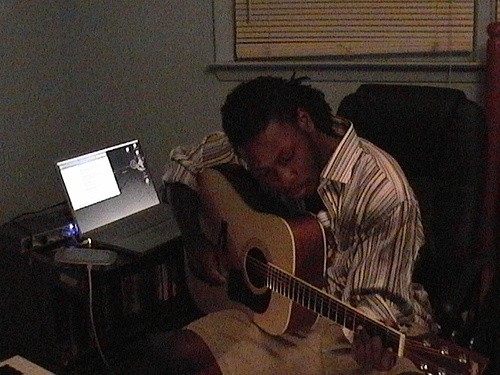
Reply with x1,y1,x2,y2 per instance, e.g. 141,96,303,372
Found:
53,138,182,256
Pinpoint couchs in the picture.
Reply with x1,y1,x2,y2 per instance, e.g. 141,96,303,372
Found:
336,82,496,375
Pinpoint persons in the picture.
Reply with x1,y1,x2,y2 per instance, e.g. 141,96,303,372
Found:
157,72,439,375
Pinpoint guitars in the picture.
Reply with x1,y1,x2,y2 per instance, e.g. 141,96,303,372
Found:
183,162,491,372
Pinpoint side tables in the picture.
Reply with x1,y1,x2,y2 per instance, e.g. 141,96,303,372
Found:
1,187,198,365
